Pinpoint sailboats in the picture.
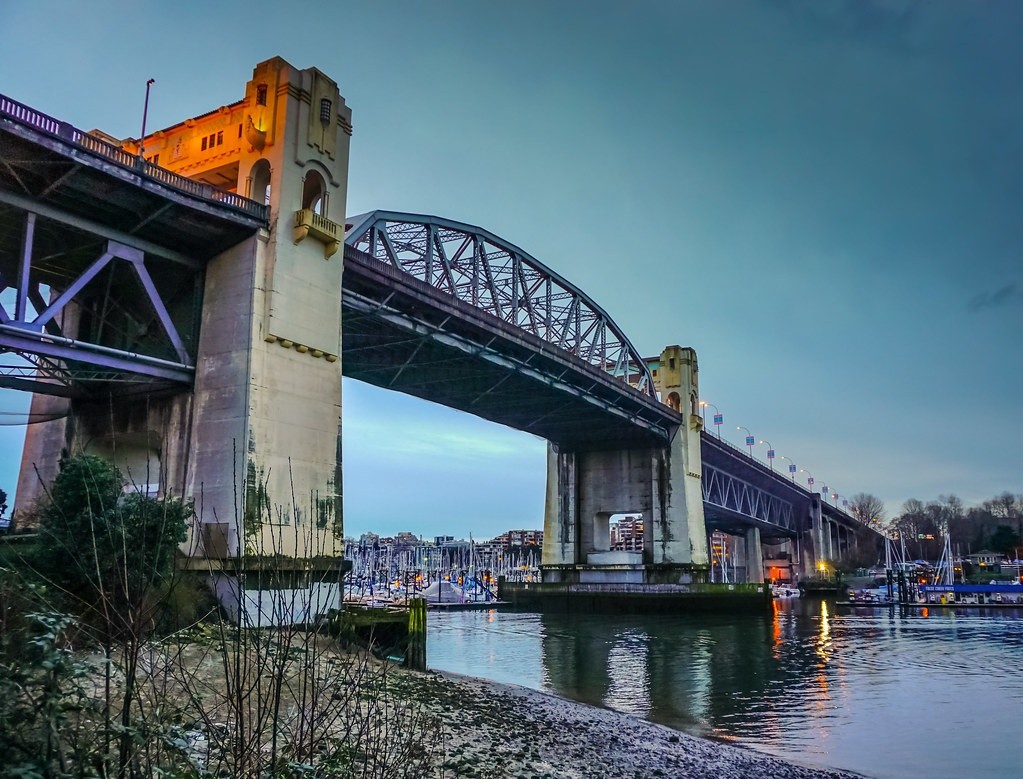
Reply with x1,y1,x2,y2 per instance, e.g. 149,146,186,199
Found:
343,532,542,609
835,529,1023,604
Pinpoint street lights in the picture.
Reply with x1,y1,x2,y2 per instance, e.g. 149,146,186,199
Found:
701,401,706,432
758,440,773,472
827,487,837,509
705,403,720,440
782,456,795,484
814,480,827,502
140,77,157,158
736,426,753,460
800,470,811,493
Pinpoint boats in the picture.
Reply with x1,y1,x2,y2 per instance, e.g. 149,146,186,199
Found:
772,583,800,599
